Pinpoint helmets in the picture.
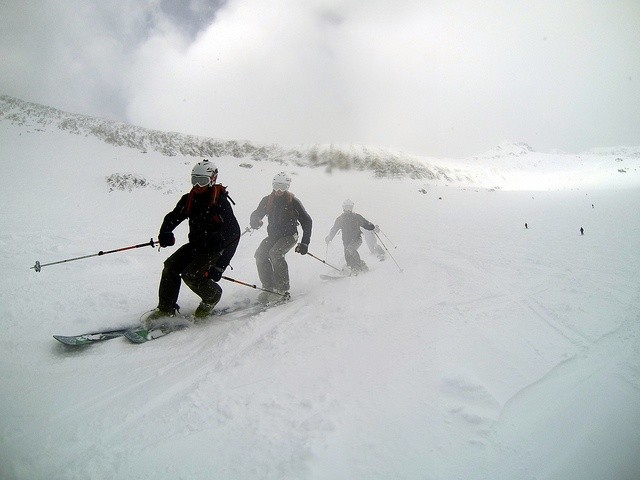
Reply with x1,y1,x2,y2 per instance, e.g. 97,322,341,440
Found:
191,159,218,178
273,172,290,184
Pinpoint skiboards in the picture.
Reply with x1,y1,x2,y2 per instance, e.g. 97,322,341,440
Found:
224,290,302,325
52,294,233,350
319,269,355,281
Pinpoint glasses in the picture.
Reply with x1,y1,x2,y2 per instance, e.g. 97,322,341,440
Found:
191,175,211,187
343,205,353,210
274,183,289,192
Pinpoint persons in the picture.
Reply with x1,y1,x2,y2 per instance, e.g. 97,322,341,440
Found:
247,171,312,304
142,158,241,325
327,200,380,274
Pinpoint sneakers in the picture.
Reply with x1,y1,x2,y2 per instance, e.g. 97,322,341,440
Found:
257,288,273,303
195,287,222,317
269,290,290,305
146,304,179,322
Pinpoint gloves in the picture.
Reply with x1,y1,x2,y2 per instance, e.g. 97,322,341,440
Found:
158,233,175,248
208,267,222,281
250,216,263,229
295,243,308,255
